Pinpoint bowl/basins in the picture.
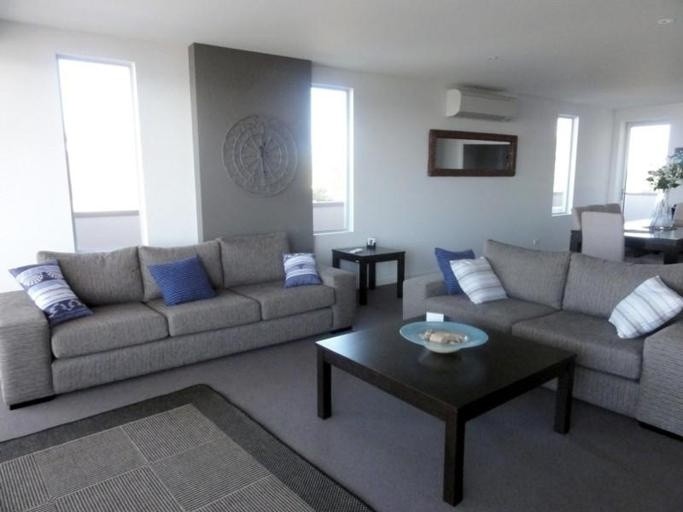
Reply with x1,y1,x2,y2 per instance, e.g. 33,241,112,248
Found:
398,320,489,354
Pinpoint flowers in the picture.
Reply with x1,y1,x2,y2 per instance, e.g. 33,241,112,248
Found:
646,145,682,215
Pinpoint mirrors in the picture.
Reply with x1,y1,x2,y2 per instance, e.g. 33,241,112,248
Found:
426,126,518,179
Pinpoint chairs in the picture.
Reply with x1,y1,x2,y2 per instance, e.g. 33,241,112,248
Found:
579,213,665,267
671,202,683,228
569,202,623,228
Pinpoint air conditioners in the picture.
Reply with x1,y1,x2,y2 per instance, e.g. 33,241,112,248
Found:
443,89,523,122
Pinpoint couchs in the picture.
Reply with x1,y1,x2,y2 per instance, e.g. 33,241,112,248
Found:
0,229,360,410
401,235,681,440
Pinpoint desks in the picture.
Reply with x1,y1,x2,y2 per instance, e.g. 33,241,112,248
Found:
327,245,406,306
568,216,682,264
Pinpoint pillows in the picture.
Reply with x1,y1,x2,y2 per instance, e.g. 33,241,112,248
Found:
278,249,320,287
5,257,93,325
434,244,477,295
605,273,682,339
447,251,510,304
144,255,221,307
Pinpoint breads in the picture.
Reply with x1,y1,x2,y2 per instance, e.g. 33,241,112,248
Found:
429,332,465,344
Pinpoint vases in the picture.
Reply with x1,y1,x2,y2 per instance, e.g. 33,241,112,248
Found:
657,190,675,228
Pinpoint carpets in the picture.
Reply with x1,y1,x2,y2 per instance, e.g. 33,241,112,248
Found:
0,382,377,510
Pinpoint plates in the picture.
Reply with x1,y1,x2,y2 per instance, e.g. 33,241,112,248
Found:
642,226,677,232
